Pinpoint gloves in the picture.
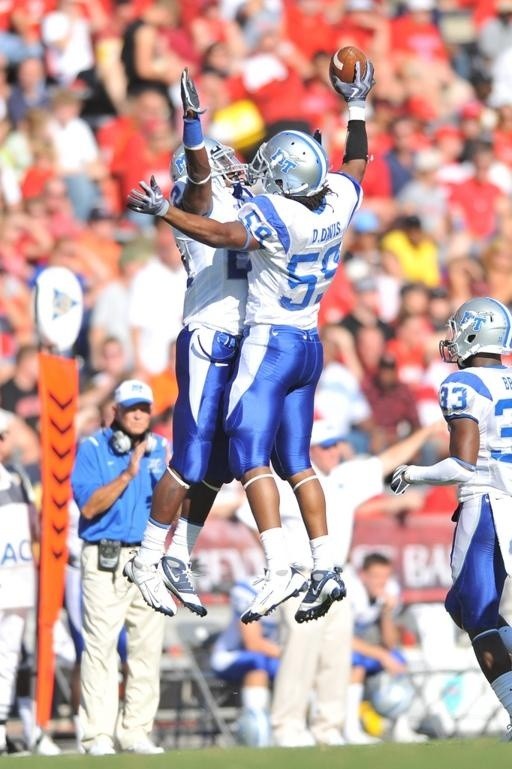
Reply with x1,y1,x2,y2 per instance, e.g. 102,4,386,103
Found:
332,59,376,122
127,177,169,218
181,67,207,119
391,465,411,495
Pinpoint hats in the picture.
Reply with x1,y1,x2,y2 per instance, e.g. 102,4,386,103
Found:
115,380,153,407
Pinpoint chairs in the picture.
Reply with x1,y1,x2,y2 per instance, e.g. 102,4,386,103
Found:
6,611,436,749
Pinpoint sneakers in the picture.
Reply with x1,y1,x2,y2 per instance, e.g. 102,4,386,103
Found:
156,558,207,616
85,735,115,755
241,567,308,624
295,570,346,622
22,727,60,757
123,556,177,617
128,740,163,755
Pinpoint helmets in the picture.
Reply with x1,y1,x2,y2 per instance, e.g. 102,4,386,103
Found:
170,136,248,189
439,297,511,367
251,130,328,198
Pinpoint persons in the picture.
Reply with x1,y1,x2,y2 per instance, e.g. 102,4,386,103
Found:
1,0,512,759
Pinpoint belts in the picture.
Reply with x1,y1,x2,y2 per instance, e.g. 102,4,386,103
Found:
90,540,140,547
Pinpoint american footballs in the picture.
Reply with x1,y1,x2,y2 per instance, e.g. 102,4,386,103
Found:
331,47,366,95
